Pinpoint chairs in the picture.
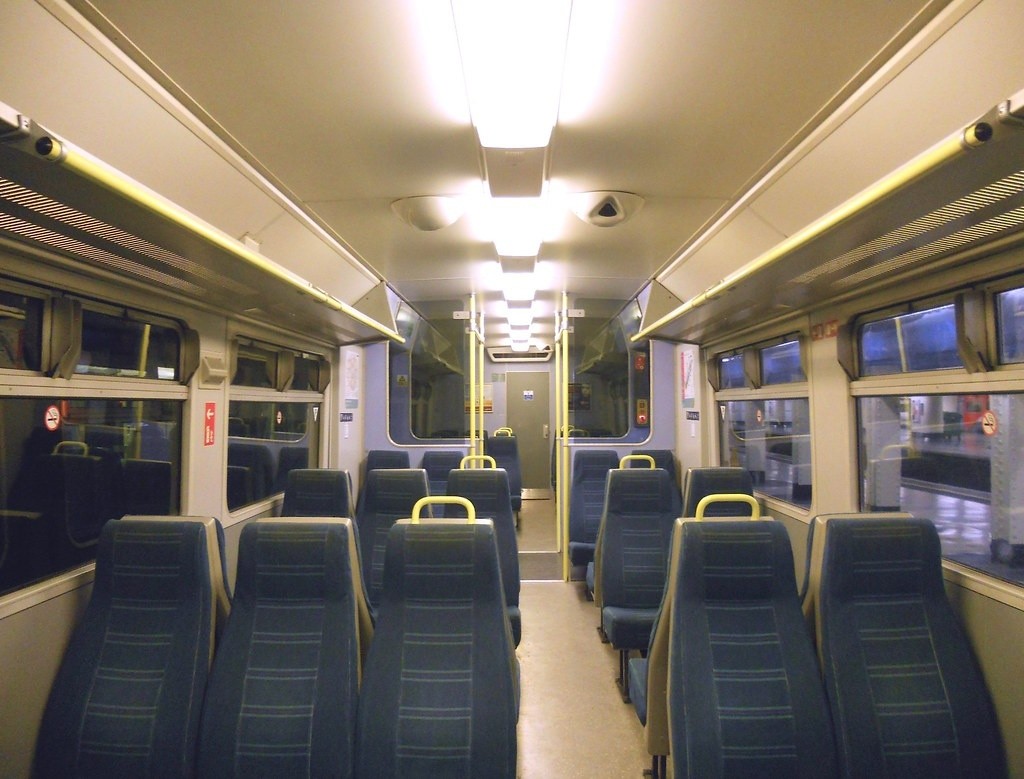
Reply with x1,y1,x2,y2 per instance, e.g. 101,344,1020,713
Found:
29,432,522,779
563,444,1010,779
0,419,310,595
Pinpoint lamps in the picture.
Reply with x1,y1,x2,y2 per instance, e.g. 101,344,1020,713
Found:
474,122,556,352
